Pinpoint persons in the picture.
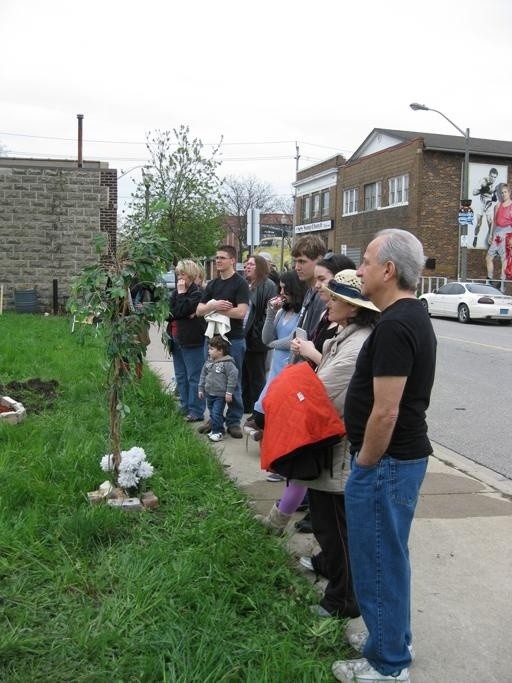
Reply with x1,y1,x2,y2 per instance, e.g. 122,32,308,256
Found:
482,183,512,291
300,267,376,620
472,167,498,248
331,228,437,682
169,235,357,533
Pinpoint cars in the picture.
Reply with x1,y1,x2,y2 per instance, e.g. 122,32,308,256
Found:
419,281,512,323
159,271,177,292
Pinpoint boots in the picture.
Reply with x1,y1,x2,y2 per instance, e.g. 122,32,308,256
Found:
252,501,295,537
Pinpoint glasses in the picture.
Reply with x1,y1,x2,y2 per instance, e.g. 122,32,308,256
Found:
322,252,341,269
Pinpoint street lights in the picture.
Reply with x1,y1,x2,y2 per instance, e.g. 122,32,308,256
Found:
278,214,290,274
410,102,472,281
116,163,154,179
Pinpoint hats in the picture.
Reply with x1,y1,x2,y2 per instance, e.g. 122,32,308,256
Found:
321,269,381,313
258,252,271,263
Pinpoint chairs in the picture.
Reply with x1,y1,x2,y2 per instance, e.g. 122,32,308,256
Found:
71,309,99,335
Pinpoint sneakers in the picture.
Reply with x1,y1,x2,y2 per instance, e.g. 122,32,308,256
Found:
473,237,477,247
294,504,313,534
197,419,243,442
331,633,414,683
308,604,332,617
299,556,314,571
266,472,287,482
180,407,204,422
